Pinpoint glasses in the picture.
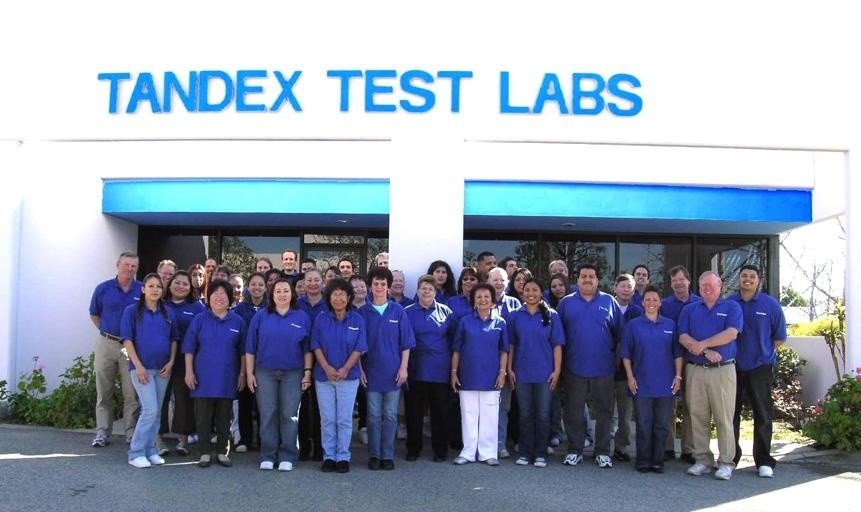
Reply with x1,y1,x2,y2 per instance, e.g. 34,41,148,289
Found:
463,278,475,281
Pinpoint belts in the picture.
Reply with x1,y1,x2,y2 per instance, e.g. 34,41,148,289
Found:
687,361,733,368
101,332,120,341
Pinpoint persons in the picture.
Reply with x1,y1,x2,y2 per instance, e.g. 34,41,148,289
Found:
87,248,792,481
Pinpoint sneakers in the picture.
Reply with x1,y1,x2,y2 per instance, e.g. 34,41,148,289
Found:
278,461,292,471
453,457,468,464
397,424,407,439
128,456,151,467
662,450,674,461
381,459,393,470
499,448,509,458
407,451,419,460
199,454,211,467
637,463,649,472
681,453,695,464
486,458,499,465
335,461,349,472
614,449,629,461
434,454,445,461
236,445,247,452
359,427,368,444
176,444,188,454
534,457,547,467
157,445,169,455
759,466,773,477
149,454,165,464
715,465,732,480
92,435,111,447
652,464,663,473
592,455,612,468
260,461,273,470
688,464,712,475
368,458,380,470
564,454,583,465
216,454,231,467
188,433,198,443
321,460,334,471
209,433,217,443
516,457,528,465
